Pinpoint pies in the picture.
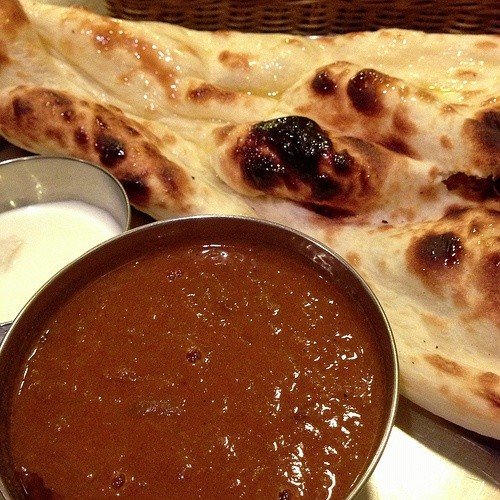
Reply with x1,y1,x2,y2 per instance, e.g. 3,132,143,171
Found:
0,0,500,438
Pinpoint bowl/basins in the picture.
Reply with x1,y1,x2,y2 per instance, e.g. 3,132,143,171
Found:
0,215,400,500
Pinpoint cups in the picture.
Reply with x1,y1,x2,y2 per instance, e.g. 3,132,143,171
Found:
0,156,131,334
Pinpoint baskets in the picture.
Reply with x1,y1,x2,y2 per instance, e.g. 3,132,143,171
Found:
104,0,500,35
